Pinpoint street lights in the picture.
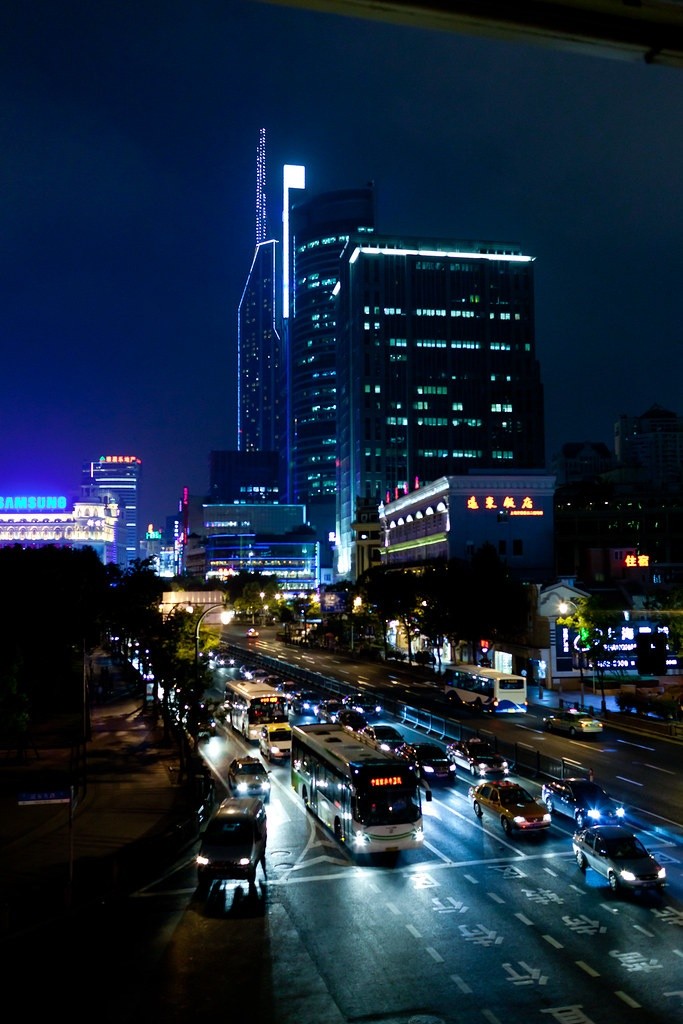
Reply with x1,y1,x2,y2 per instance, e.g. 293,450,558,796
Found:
161,600,194,716
559,601,584,710
192,604,233,759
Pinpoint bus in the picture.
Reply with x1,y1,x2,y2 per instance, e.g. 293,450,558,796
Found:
291,725,431,854
445,664,528,716
225,680,290,741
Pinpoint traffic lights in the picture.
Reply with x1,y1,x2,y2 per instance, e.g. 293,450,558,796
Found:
579,627,593,650
478,637,493,660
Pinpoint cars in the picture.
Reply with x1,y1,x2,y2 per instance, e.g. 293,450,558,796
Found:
468,779,550,838
395,742,456,781
335,708,369,733
358,726,409,758
316,700,344,723
446,737,509,776
542,777,626,828
342,694,384,717
290,689,324,714
543,708,603,740
259,724,292,759
197,627,301,701
573,824,665,895
228,756,271,800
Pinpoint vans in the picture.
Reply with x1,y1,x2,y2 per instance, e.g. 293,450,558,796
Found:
196,796,268,888
